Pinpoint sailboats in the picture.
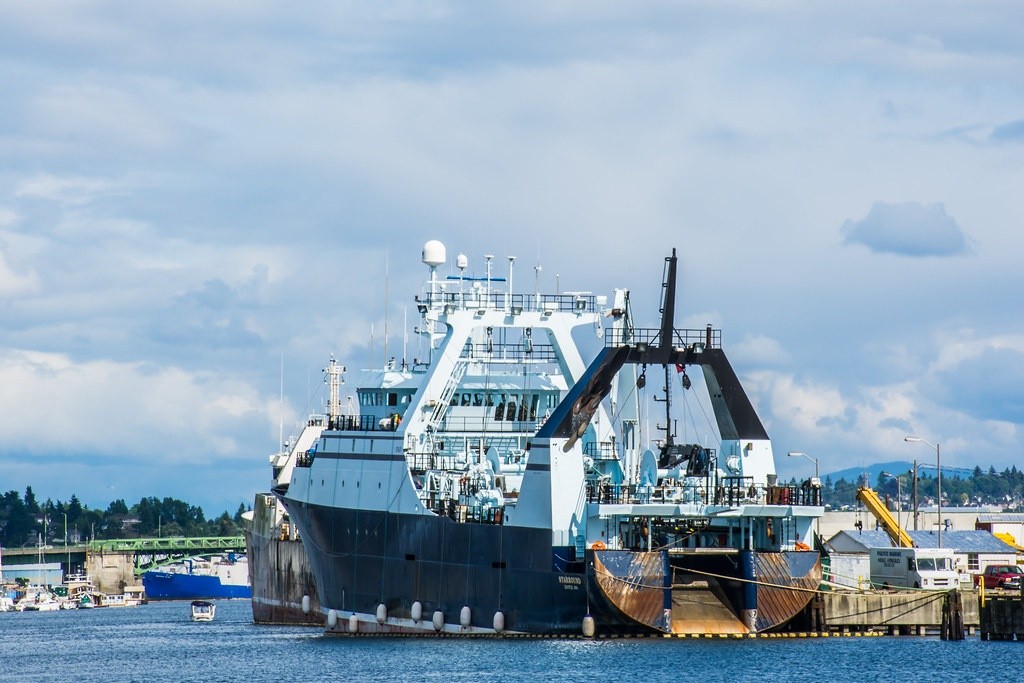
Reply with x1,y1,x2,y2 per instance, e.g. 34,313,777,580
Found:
0,532,148,612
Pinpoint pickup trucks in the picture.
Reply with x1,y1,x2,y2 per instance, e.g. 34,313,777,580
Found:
974,564,1024,590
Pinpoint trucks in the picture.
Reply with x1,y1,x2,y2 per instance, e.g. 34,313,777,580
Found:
869,547,961,592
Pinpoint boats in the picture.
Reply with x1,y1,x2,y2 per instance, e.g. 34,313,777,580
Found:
270,238,824,639
142,549,252,601
191,599,216,622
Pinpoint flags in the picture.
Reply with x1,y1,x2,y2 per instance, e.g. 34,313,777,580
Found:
676,363,683,374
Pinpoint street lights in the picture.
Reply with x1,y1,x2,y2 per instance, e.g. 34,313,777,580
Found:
904,437,942,548
61,513,67,545
884,473,901,546
788,452,818,478
